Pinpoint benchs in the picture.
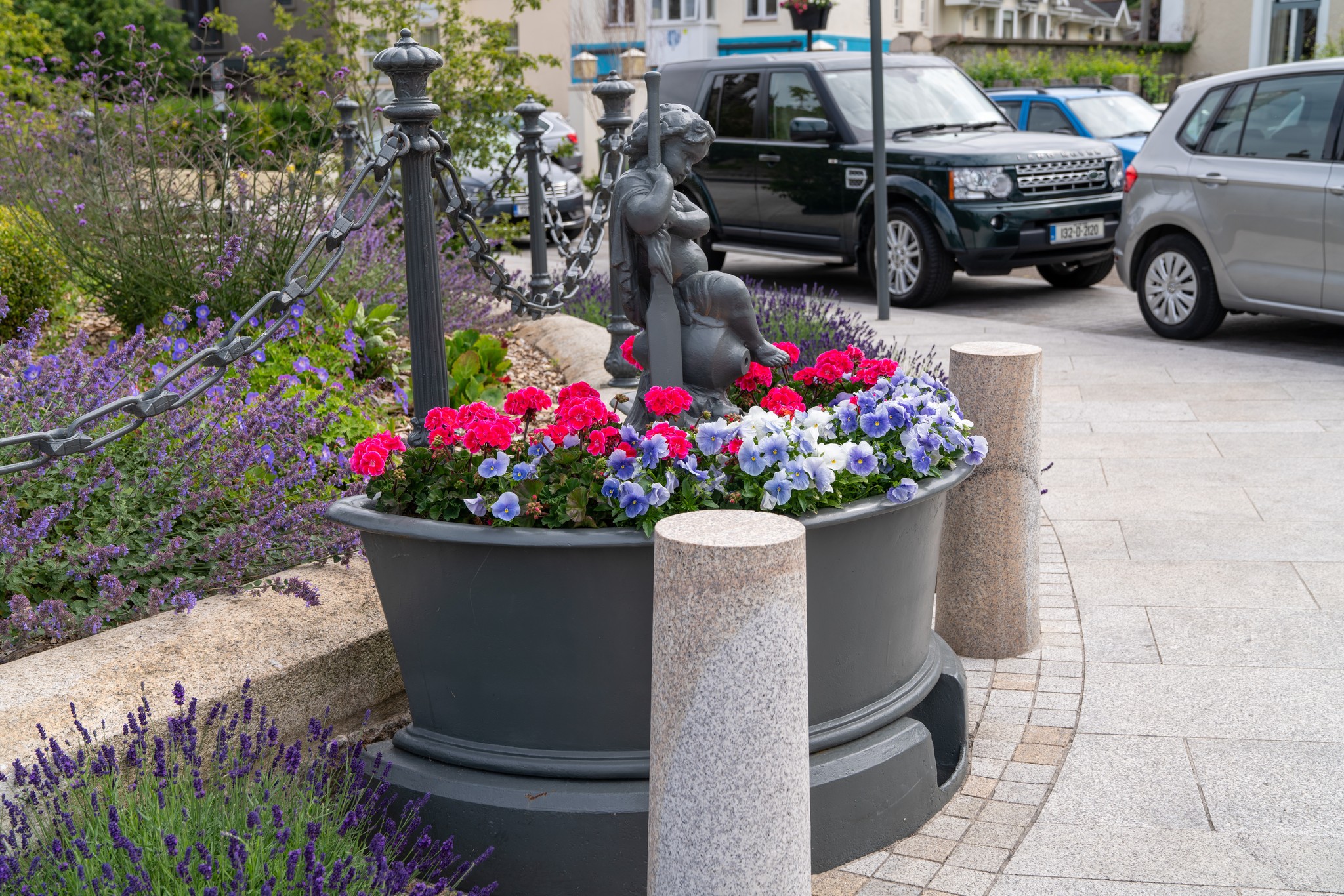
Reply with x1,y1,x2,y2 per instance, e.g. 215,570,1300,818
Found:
1218,124,1313,160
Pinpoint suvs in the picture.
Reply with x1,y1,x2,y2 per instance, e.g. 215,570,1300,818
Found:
657,50,1126,310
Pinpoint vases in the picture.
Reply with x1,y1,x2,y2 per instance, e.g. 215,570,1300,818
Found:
327,463,976,783
788,6,828,29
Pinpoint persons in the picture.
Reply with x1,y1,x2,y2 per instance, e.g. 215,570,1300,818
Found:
611,102,789,369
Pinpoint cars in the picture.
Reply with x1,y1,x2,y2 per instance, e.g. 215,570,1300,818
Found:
984,82,1163,178
359,110,596,248
1111,52,1344,342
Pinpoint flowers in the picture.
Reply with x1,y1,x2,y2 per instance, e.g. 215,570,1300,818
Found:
780,0,837,14
348,330,988,538
